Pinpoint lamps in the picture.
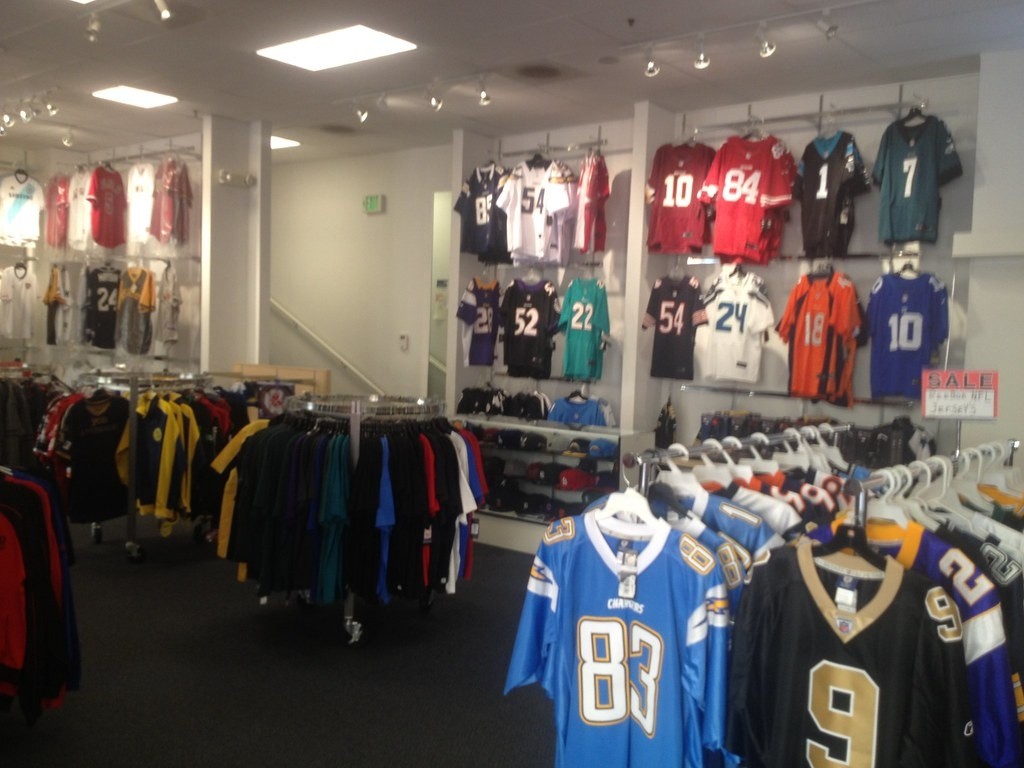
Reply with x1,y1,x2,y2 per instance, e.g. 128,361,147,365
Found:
1,12,102,149
644,45,660,77
692,32,711,69
425,92,444,112
753,18,777,58
814,8,839,40
479,81,491,106
154,0,171,21
351,103,369,123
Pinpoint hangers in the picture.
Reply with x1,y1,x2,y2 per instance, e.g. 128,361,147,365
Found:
0,89,1024,599
